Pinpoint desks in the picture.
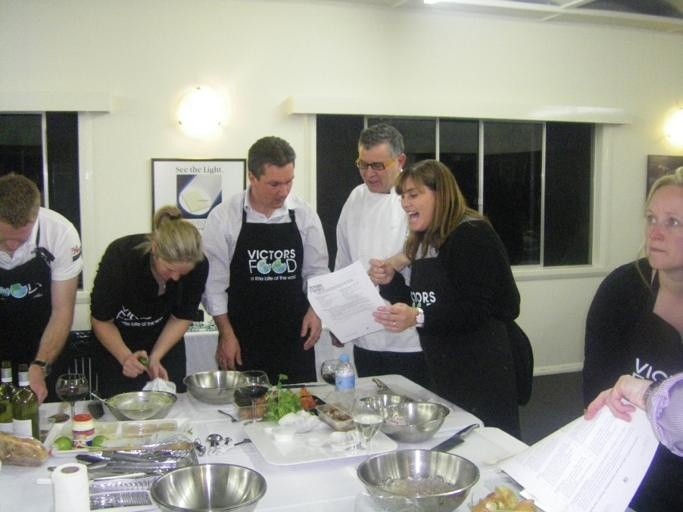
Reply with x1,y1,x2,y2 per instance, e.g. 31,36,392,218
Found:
185,327,354,379
0,374,533,512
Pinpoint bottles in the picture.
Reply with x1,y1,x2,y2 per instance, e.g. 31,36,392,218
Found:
72,414,95,450
336,356,357,408
0,360,40,443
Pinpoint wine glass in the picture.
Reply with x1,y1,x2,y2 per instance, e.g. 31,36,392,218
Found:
320,359,345,405
56,374,89,421
236,370,270,423
352,397,383,449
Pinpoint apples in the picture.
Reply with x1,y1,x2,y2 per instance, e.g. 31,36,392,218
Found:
54,437,72,451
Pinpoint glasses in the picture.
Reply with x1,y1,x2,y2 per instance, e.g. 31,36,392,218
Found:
353,156,401,172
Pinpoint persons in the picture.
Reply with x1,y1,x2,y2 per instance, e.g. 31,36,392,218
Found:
90,205,209,399
0,173,83,405
367,159,521,440
582,166,683,512
201,137,328,386
586,372,683,457
329,123,430,389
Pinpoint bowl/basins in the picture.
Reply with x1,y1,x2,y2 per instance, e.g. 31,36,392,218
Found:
147,464,269,510
104,391,177,422
374,402,450,444
183,371,244,405
360,395,415,415
356,447,481,507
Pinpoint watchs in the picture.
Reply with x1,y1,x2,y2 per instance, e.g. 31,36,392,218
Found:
414,308,424,327
30,360,52,377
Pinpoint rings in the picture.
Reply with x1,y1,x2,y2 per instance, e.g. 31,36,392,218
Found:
393,322,395,327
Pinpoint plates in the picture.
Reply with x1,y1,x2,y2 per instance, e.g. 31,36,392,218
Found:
241,419,395,467
44,418,196,458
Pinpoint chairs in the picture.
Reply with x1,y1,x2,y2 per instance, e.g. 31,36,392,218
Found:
45,330,99,400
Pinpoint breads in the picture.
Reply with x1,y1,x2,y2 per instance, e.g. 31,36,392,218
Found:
0,430,48,466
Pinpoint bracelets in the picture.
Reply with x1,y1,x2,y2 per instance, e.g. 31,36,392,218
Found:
643,377,666,405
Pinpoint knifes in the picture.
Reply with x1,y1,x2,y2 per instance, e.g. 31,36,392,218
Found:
429,422,481,451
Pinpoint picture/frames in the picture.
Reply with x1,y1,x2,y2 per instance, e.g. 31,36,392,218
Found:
151,157,246,235
